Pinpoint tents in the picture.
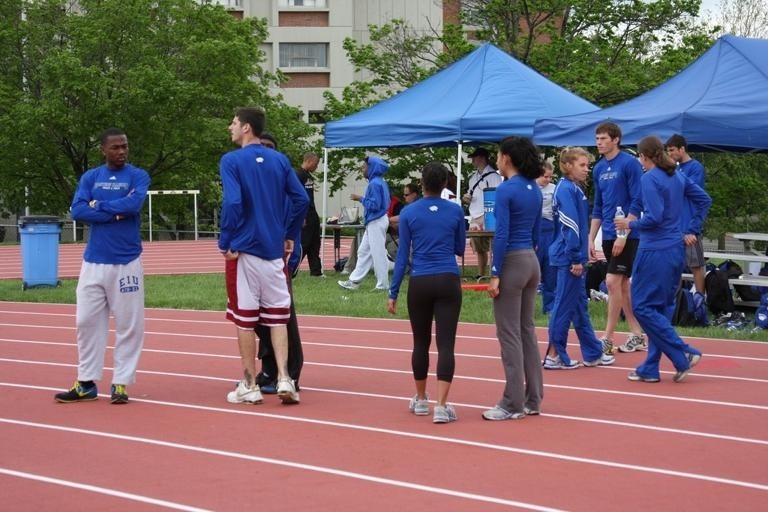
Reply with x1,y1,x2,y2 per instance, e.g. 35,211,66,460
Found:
322,43,768,278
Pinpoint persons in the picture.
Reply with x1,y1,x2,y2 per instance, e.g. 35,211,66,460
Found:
387,161,467,423
295,150,321,276
468,122,712,420
55,126,151,404
217,106,310,405
336,154,392,293
255,133,304,394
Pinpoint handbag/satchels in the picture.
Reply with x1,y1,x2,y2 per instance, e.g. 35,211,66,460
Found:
464,208,470,233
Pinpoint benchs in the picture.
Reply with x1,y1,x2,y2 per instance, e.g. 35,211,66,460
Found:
680,231,767,317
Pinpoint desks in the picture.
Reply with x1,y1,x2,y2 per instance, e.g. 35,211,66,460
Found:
320,223,494,286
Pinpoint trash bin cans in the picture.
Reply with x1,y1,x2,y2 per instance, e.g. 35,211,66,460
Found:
18,214,62,292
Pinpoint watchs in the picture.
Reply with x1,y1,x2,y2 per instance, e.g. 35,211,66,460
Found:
88,198,96,209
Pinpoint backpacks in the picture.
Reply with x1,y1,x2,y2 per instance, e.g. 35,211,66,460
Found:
674,288,710,328
585,258,608,299
703,259,743,317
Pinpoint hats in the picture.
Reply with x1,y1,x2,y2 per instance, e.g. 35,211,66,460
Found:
467,146,491,158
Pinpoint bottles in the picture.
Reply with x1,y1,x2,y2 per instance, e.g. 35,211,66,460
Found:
614,206,628,240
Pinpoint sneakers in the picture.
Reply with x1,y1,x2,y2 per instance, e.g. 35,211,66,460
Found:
109,384,129,404
618,334,647,352
627,368,660,382
226,379,265,406
672,350,702,383
583,353,615,367
368,286,391,292
543,353,580,370
522,406,540,415
259,371,300,393
482,405,527,420
275,377,300,404
596,336,615,354
337,279,360,291
432,405,458,423
53,380,98,403
408,393,430,417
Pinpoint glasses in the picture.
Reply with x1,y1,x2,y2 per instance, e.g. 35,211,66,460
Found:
402,193,411,197
